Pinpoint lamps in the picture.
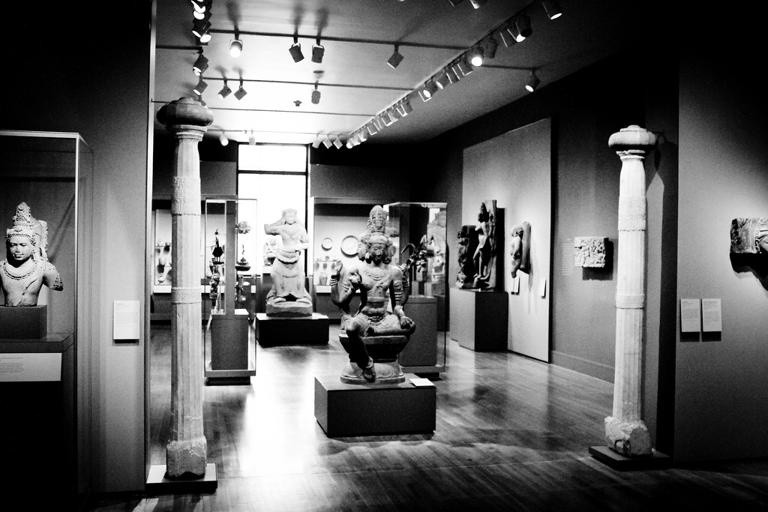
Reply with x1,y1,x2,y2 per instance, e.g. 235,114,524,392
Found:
312,87,320,105
191,21,212,40
194,77,207,95
192,64,210,75
418,1,564,101
228,40,243,58
233,81,247,100
386,46,404,70
193,55,208,72
192,1,211,27
249,135,257,147
200,30,212,44
311,39,325,63
313,98,412,152
217,80,232,98
219,131,228,147
288,36,304,63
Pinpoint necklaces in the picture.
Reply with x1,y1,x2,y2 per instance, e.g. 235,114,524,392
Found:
3,258,38,281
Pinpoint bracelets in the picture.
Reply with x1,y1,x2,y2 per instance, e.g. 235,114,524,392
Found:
330,279,337,286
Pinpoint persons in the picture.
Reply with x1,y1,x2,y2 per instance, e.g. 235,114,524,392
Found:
265,208,313,304
426,211,446,298
472,202,491,279
510,227,523,277
457,224,472,271
1,225,64,307
330,235,415,380
358,207,393,264
